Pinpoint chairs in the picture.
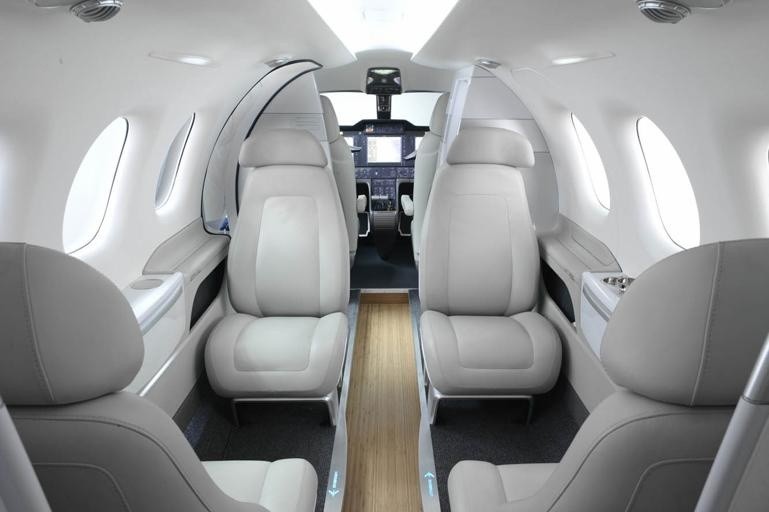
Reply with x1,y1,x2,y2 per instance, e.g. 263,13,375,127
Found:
418,127,564,425
447,237,769,512
202,128,350,428
320,94,367,267
0,240,319,512
402,92,450,265
